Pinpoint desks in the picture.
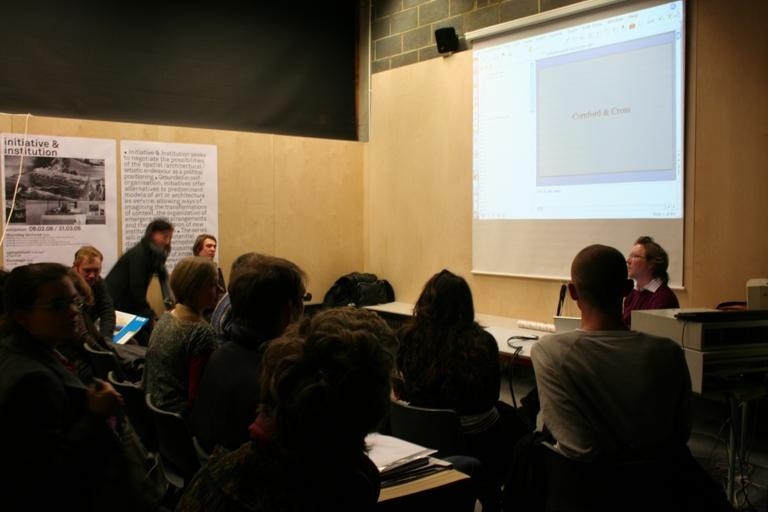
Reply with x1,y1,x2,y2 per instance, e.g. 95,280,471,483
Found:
360,302,556,363
363,436,470,512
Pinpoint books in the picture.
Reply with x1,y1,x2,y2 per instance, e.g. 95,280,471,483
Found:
361,432,452,489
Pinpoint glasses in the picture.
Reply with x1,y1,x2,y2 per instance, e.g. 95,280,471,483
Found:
22,297,83,312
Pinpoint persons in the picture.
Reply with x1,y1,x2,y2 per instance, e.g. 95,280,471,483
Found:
176,306,400,511
0,262,123,512
530,245,694,510
193,233,226,322
622,237,680,325
395,269,529,512
104,216,174,347
182,257,312,486
73,247,120,358
139,260,224,464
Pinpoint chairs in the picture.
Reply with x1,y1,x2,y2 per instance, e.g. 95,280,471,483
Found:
143,395,183,454
108,371,149,430
82,345,120,380
